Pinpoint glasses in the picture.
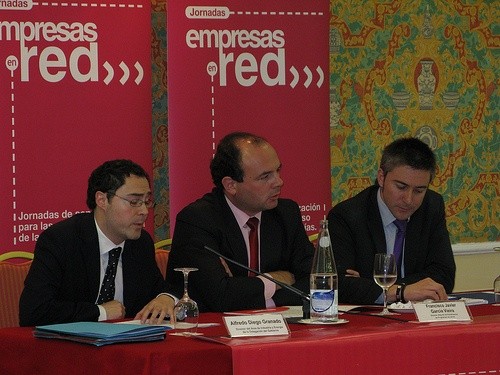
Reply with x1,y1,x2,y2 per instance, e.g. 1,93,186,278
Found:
113,193,156,209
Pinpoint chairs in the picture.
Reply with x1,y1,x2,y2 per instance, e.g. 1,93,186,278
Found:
0,251,34,328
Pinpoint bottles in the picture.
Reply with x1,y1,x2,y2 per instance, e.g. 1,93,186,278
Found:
309,221,339,323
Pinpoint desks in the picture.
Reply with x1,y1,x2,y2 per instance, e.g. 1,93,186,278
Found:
0,290,500,375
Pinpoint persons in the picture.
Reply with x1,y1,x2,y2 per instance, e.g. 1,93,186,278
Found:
165,133,328,312
323,138,455,304
19,160,186,325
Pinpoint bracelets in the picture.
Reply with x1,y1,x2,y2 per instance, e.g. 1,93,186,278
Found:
396,284,401,303
400,283,408,304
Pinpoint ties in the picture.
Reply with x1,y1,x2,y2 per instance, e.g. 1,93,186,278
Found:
385,218,408,283
246,217,260,278
96,246,122,305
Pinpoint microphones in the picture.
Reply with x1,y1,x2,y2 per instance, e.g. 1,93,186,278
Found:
190,239,311,302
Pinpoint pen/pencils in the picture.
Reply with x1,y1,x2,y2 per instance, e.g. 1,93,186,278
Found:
448,296,461,300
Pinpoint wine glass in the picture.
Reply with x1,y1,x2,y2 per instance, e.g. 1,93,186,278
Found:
373,253,401,315
493,246,500,303
172,267,199,336
